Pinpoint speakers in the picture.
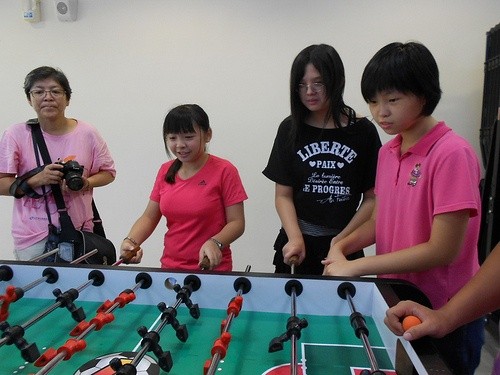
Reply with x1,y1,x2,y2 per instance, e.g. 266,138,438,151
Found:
55,0,77,21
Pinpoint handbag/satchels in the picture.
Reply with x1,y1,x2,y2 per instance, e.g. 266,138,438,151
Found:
38,223,116,266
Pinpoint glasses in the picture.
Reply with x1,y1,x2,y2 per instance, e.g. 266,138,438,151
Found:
298,83,325,95
28,88,66,99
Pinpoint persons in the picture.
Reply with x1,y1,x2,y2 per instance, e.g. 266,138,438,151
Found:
384,241,500,341
262,44,382,274
0,66,116,261
121,105,248,271
322,42,485,375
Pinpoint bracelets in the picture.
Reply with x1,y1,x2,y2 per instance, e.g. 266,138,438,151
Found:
210,238,224,249
124,237,136,244
87,182,89,187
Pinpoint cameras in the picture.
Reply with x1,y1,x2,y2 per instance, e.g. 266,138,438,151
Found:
55,160,84,191
